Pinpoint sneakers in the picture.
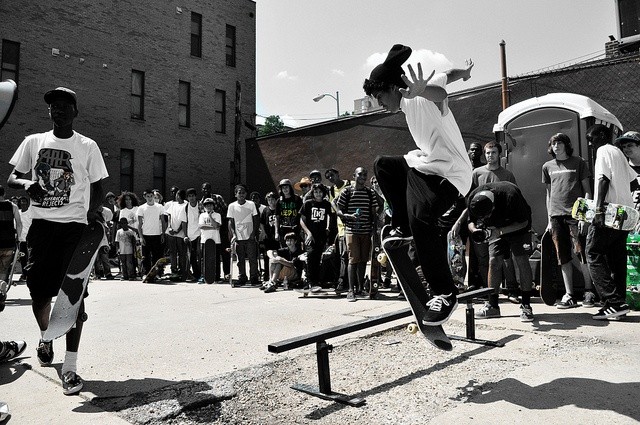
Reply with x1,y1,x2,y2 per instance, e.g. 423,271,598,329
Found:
592,301,630,319
36,336,54,366
129,276,134,281
62,371,84,393
346,289,356,302
555,292,578,309
224,274,231,283
582,290,596,307
519,302,536,322
382,276,392,289
311,285,322,293
265,285,276,293
0,401,9,424
473,301,501,318
508,290,520,303
422,289,459,326
383,224,415,250
106,273,114,279
335,279,344,293
0,338,27,361
250,278,264,287
234,273,248,287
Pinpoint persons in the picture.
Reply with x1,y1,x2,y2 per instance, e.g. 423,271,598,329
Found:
0,186,23,312
613,131,640,235
184,188,206,282
337,166,379,299
198,182,229,278
370,176,402,289
166,190,187,281
198,198,224,284
542,132,595,309
469,141,518,288
468,180,534,322
164,186,188,203
467,142,486,170
300,184,332,288
250,191,271,281
309,169,322,184
153,189,163,204
96,193,122,280
117,191,147,279
152,190,166,206
0,79,26,425
260,191,280,282
260,231,302,293
11,196,20,207
275,179,302,248
294,177,312,200
137,190,166,280
226,184,260,286
325,168,356,290
7,86,109,395
586,124,640,322
363,44,473,327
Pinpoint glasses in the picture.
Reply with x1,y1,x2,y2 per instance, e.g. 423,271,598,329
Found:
47,101,77,113
300,183,309,187
311,175,320,182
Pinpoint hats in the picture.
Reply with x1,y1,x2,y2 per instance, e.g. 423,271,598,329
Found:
105,191,116,200
614,130,640,143
309,169,321,176
43,85,78,109
278,178,291,187
370,43,412,87
468,197,492,219
203,198,215,204
293,176,313,190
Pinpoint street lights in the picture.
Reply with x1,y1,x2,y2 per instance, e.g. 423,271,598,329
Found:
312,90,339,119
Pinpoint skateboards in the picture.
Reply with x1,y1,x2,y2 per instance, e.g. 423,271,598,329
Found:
540,230,558,306
447,230,466,294
624,233,640,309
204,239,216,284
571,197,639,231
226,241,234,287
368,234,382,300
377,225,452,352
42,220,110,342
6,248,25,293
294,289,344,297
143,258,166,283
